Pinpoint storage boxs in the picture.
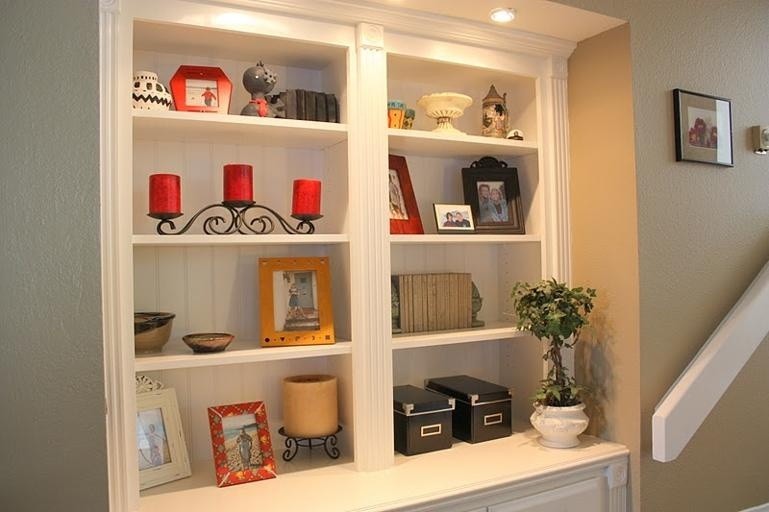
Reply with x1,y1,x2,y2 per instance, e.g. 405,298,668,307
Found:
393,384,456,455
425,375,512,444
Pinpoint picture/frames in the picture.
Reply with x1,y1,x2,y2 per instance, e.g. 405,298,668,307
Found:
462,157,525,234
136,375,193,491
433,203,477,234
207,402,277,488
258,257,336,347
673,88,734,167
170,65,233,114
390,154,424,234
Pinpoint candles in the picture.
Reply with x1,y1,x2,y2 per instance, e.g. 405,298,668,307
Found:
223,165,253,202
282,374,338,438
292,179,321,215
148,174,180,212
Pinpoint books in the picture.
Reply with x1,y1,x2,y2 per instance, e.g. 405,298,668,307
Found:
390,272,473,334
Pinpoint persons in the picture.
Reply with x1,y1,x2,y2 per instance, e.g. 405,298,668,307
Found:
289,283,310,320
389,174,405,218
237,428,252,470
455,212,470,227
478,184,508,222
202,87,216,106
689,118,717,148
144,424,165,465
443,213,458,227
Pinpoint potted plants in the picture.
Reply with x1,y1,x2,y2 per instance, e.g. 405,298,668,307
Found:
511,280,596,449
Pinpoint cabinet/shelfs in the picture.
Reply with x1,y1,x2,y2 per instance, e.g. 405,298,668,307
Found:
97,1,631,510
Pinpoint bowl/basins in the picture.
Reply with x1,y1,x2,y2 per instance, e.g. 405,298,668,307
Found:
133,311,175,357
182,332,234,352
